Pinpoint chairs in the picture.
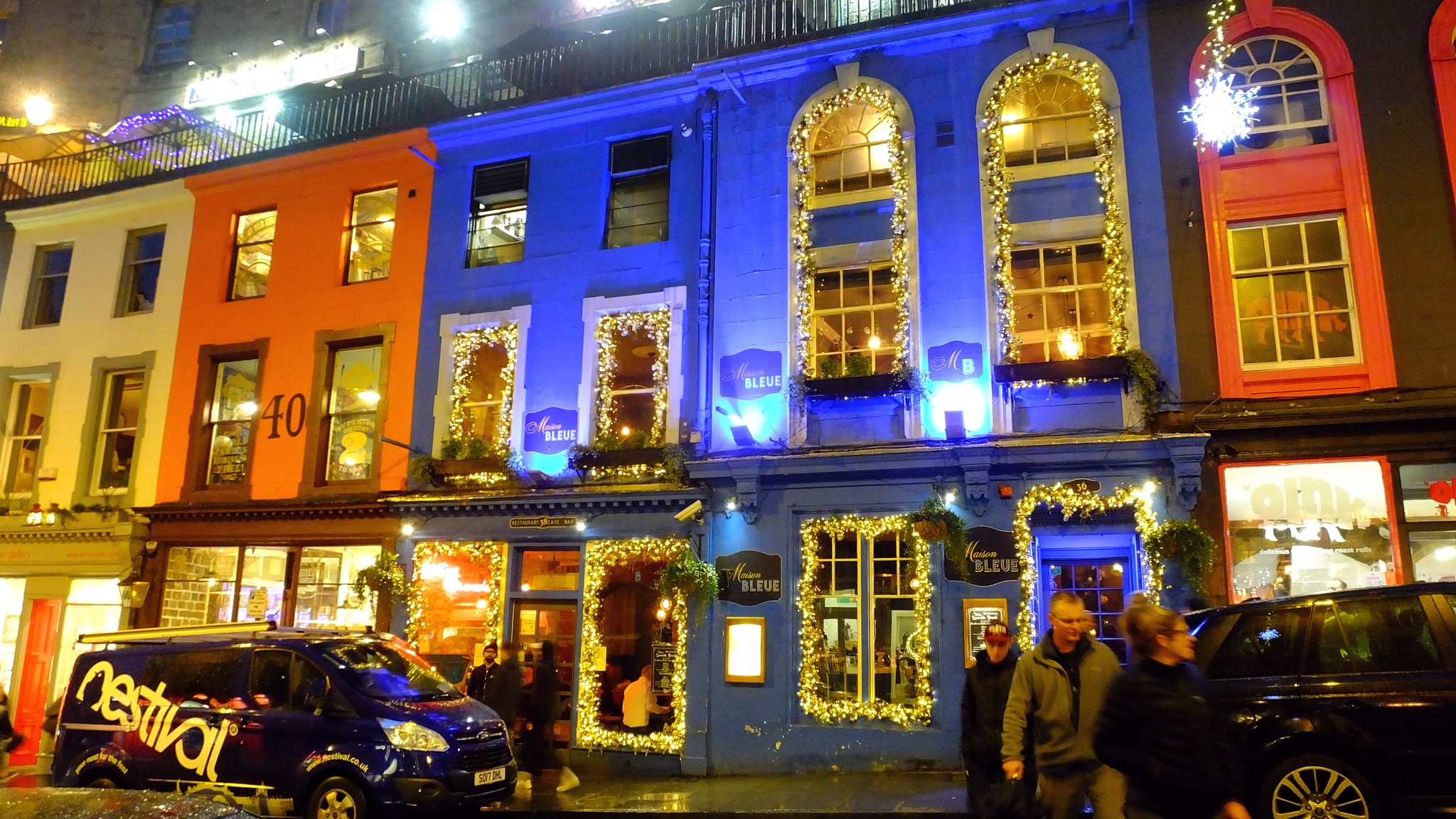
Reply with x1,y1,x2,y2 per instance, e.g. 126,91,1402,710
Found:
824,658,856,705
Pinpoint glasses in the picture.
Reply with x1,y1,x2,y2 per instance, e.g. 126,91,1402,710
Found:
1161,628,1193,635
1049,612,1083,624
485,650,495,653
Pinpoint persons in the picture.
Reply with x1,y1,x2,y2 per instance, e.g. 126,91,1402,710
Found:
1089,593,1252,819
961,622,1038,818
467,641,581,808
40,687,67,736
622,664,670,734
0,683,23,756
1398,609,1442,671
1001,592,1130,819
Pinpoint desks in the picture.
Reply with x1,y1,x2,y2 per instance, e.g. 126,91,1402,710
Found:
844,666,892,674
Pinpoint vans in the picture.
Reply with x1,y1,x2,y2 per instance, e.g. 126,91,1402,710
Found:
51,621,518,819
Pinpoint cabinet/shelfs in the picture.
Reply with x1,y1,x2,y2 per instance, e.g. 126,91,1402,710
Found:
891,610,917,707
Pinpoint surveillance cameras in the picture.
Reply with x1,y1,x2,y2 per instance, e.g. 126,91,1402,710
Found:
674,500,702,523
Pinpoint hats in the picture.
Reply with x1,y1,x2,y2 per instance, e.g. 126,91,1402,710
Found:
984,621,1013,644
483,639,498,657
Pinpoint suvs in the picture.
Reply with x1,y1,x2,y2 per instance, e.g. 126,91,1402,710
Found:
1182,580,1456,819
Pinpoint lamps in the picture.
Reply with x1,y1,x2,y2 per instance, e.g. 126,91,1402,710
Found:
117,552,151,609
358,348,380,405
868,291,881,352
1055,276,1079,360
1176,0,1260,154
237,360,258,415
46,503,61,525
846,296,854,334
26,503,43,526
198,549,222,586
864,327,871,334
376,191,395,229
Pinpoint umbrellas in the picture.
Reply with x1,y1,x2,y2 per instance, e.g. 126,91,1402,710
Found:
272,0,814,137
103,104,244,170
1,128,154,191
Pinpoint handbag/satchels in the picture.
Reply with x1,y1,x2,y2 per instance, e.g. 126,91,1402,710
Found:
976,777,1036,819
